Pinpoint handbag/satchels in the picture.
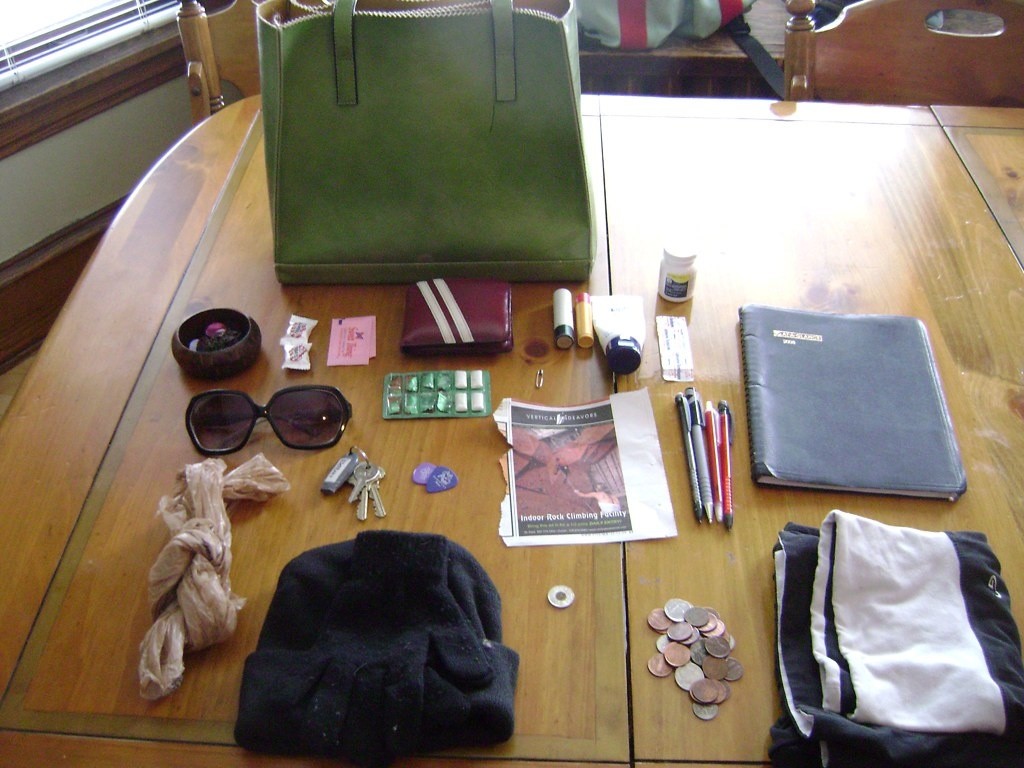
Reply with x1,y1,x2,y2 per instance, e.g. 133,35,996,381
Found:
255,0,594,284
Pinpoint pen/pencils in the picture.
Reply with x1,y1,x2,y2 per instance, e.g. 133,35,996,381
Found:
674,386,739,534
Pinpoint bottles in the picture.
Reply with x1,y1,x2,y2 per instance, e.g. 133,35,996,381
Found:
659,247,696,302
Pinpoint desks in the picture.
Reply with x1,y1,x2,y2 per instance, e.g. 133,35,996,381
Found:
0,94,1024,768
579,0,787,99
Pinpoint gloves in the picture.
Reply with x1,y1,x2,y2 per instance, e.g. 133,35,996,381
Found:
277,530,497,768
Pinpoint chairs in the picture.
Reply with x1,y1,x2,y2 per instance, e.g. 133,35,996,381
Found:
783,0,1024,108
176,1,261,129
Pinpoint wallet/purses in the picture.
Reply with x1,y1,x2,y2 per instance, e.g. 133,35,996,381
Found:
399,276,514,356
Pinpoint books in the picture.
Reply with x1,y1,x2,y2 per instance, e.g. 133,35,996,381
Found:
739,304,967,502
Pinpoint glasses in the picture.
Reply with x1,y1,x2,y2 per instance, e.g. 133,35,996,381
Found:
185,384,352,456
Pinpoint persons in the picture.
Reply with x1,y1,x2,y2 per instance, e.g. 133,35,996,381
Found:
575,483,621,513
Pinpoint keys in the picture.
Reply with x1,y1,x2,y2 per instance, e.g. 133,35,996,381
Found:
320,446,386,520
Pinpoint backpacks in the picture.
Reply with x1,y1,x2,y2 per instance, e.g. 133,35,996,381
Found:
575,0,757,53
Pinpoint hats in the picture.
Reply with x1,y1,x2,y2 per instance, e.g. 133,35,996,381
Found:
233,531,520,752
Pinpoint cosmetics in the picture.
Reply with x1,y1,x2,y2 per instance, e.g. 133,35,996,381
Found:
553,289,645,374
